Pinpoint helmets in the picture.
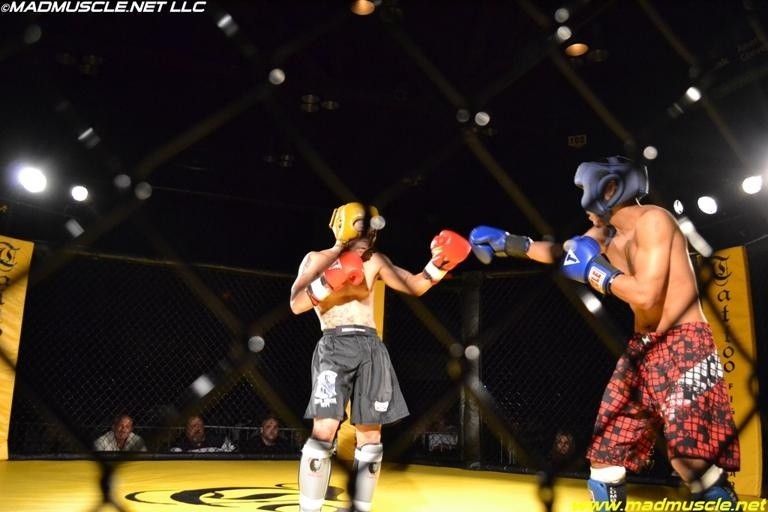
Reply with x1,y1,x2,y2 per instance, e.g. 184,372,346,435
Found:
329,201,382,248
574,155,650,216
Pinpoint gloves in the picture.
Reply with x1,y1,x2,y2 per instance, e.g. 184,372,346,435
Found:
469,225,530,265
561,236,622,298
305,253,365,306
424,228,471,285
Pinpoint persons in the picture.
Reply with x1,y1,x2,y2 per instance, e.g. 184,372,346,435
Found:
93,415,147,452
537,431,588,478
239,415,294,455
169,417,216,452
289,201,473,512
470,153,745,512
